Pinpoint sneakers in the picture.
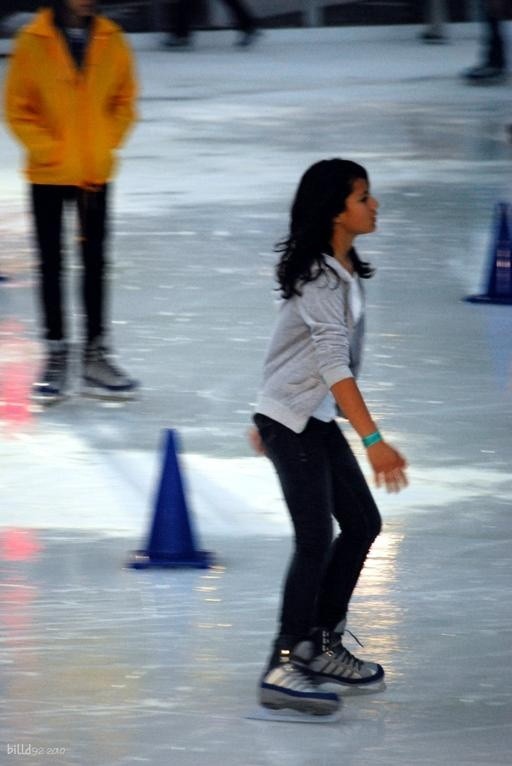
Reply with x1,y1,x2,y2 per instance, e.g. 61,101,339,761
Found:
79,336,138,392
33,337,70,395
259,625,343,713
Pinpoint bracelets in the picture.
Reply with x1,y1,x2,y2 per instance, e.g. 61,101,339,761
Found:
361,431,382,449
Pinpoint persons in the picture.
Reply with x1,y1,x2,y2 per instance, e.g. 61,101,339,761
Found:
455,0,510,83
156,1,262,54
414,0,451,45
246,157,410,715
1,1,140,405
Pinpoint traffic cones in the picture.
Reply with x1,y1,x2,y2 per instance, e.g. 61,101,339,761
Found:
125,426,210,567
462,201,512,307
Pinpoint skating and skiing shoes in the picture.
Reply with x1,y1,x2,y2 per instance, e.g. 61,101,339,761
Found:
311,618,387,685
